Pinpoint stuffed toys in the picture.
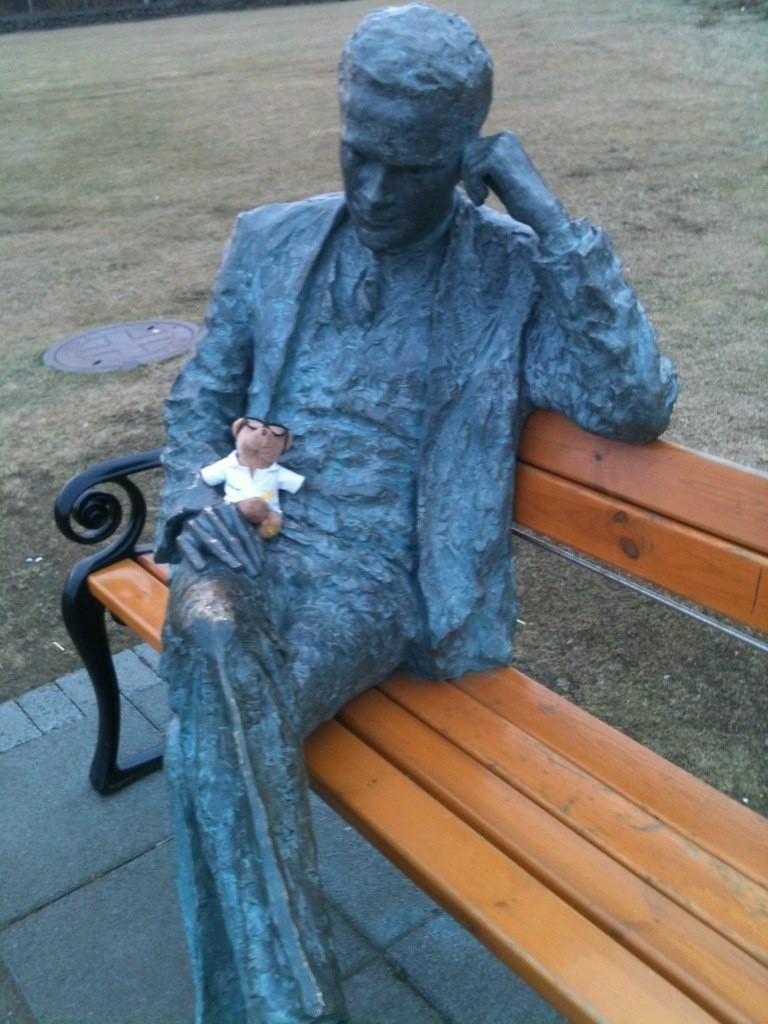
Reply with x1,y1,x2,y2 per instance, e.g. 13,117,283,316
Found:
200,416,306,537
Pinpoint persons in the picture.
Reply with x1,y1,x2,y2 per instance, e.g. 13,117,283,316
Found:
153,2,680,1024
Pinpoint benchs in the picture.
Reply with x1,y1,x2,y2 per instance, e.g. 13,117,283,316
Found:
55,410,768,1023
